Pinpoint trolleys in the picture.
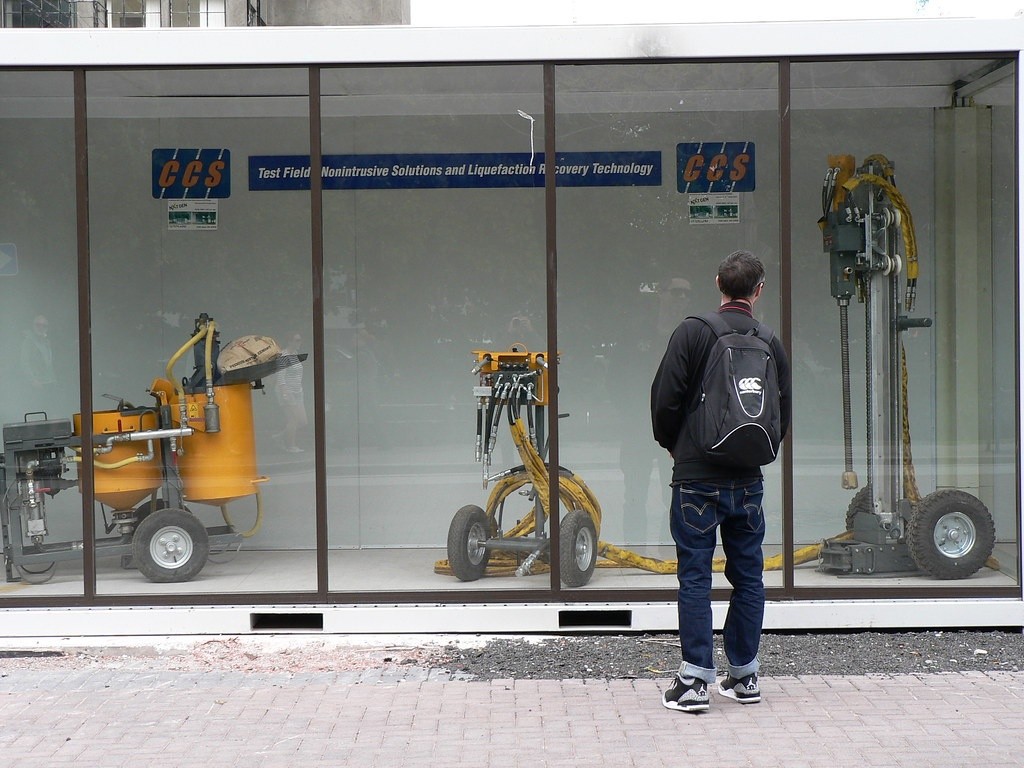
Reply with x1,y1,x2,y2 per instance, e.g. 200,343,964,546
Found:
0,312,311,586
814,153,997,578
445,349,598,588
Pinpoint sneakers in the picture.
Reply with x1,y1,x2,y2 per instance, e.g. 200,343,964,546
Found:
718,673,761,703
662,673,710,711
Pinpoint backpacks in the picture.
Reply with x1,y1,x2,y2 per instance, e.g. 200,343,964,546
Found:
684,312,780,465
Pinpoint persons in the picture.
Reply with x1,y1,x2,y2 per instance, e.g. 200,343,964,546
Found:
652,250,792,711
